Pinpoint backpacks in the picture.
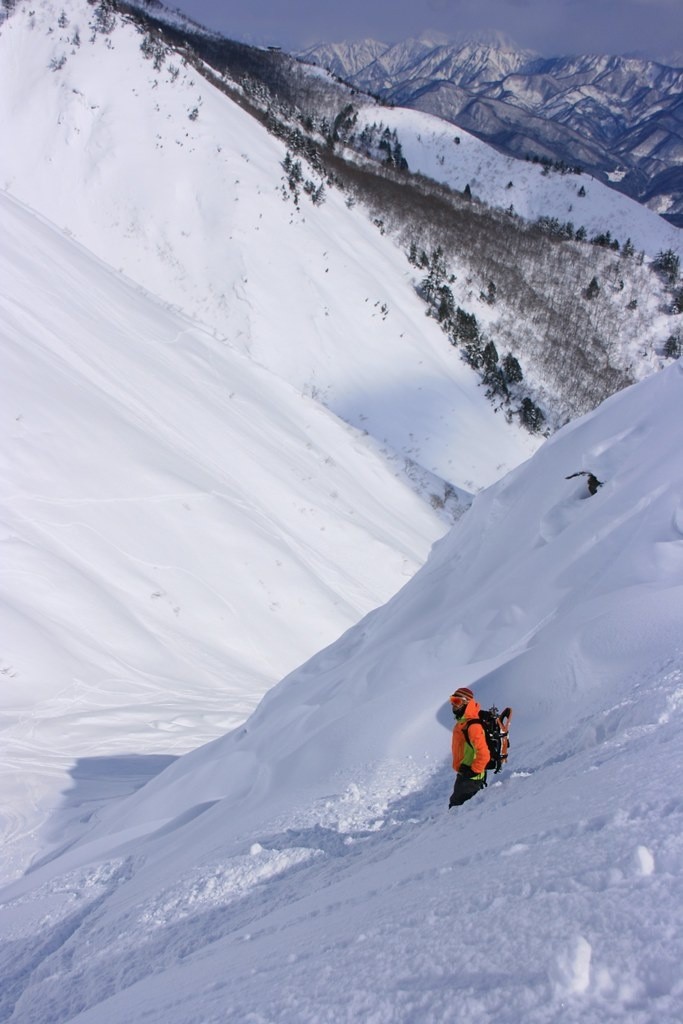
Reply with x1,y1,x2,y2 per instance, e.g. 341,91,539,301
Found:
463,707,512,774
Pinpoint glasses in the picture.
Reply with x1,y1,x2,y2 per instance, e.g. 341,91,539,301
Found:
450,696,466,705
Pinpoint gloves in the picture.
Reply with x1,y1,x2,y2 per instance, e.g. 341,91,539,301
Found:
459,764,479,778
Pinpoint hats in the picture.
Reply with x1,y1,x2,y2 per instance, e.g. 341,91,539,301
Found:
452,688,473,701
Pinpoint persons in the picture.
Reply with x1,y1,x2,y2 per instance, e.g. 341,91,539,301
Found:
449,687,491,809
